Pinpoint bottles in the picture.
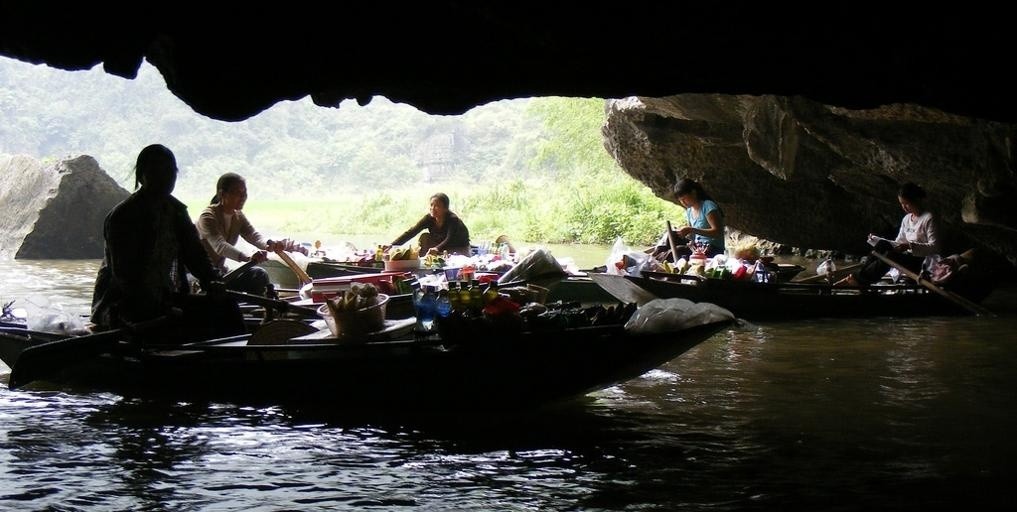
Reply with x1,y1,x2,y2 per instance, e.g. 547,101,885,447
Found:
457,280,471,312
415,285,437,331
374,245,383,270
483,280,499,304
468,280,483,310
445,281,460,308
436,288,449,317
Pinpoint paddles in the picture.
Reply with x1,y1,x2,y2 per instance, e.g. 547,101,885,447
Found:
8,250,249,391
869,248,996,315
666,221,688,270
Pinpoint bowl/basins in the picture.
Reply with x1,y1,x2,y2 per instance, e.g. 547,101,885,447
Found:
383,259,420,270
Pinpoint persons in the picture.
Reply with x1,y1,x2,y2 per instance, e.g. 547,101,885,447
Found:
381,192,472,256
843,181,945,285
616,178,724,263
88,143,226,340
195,171,285,295
